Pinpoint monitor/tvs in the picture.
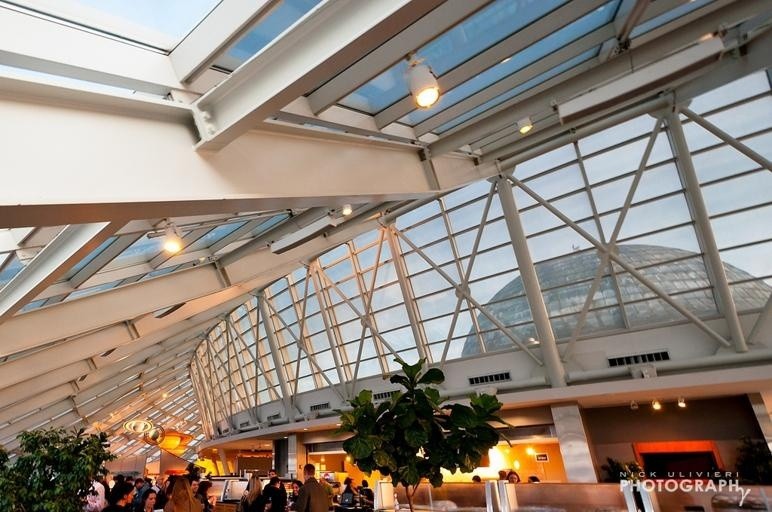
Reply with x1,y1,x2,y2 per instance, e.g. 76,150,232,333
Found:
223,480,249,501
259,477,271,495
207,480,227,503
320,472,334,482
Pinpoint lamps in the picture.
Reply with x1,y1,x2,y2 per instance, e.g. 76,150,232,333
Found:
517,117,533,134
404,55,441,110
342,204,352,215
653,398,661,410
153,218,183,253
678,396,686,408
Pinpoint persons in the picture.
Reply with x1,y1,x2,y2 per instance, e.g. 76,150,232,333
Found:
284,479,304,512
360,480,374,507
497,470,507,479
626,464,642,483
294,463,330,512
319,476,336,511
76,462,287,511
506,470,520,483
340,476,357,507
472,475,481,482
527,475,540,483
